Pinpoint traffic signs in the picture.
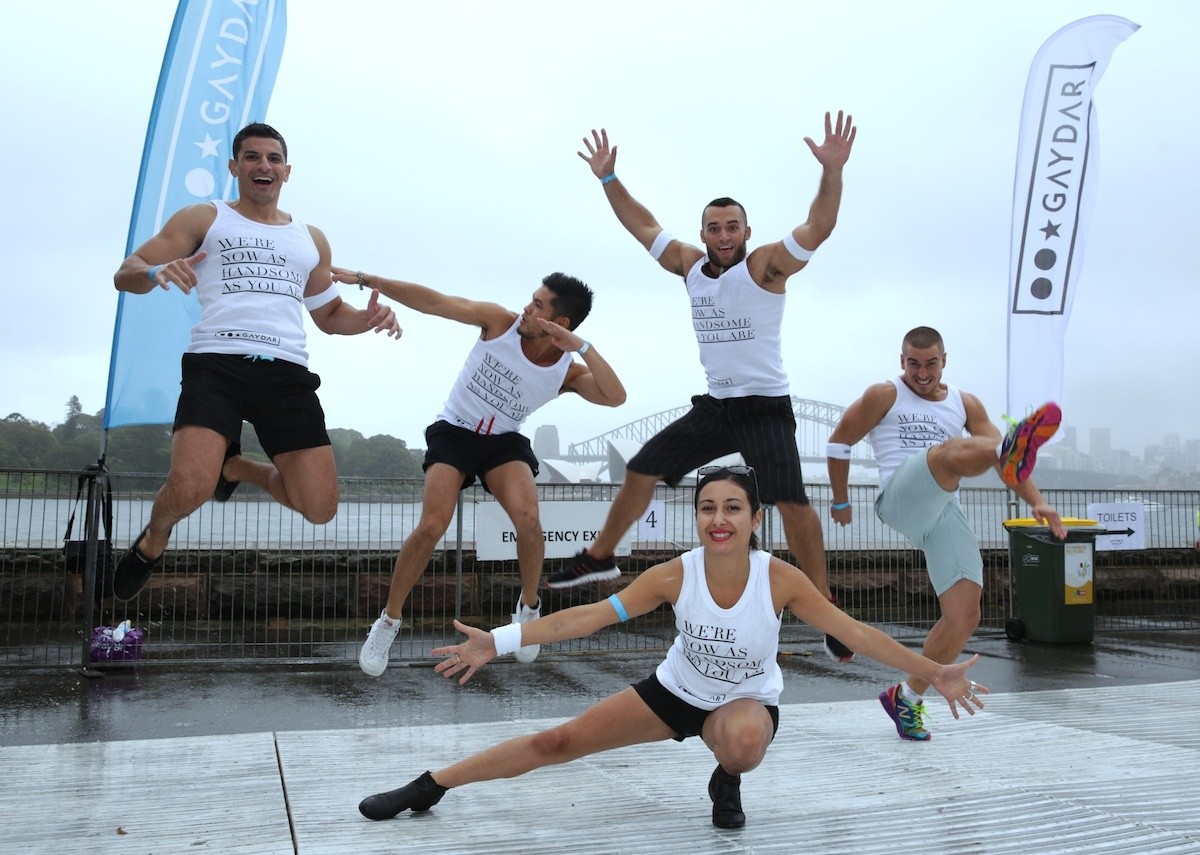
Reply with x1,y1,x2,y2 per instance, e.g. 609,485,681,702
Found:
1086,502,1146,552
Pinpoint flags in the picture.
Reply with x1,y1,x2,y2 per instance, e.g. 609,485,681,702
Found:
1009,16,1142,450
108,0,287,427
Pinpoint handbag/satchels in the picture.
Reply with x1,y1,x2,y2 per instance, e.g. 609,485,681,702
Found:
92,621,143,661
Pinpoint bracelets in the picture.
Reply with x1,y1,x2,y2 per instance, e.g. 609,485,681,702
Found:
831,501,849,509
600,173,617,184
149,265,164,286
491,623,522,655
578,341,590,355
358,271,365,290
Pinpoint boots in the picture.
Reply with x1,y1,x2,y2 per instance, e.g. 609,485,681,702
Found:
709,764,746,828
359,771,449,821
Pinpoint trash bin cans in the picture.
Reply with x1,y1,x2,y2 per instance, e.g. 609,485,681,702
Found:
1001,517,1107,645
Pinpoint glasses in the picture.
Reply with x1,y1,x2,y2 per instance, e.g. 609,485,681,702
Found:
696,465,760,508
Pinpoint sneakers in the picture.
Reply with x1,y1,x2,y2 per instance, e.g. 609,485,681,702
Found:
544,549,621,589
878,683,932,741
996,400,1061,487
359,607,402,677
214,448,241,502
823,631,856,664
111,523,170,603
510,590,541,663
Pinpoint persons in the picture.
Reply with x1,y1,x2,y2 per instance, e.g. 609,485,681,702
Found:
112,124,403,602
827,326,1067,742
331,266,627,676
549,110,856,665
359,466,990,828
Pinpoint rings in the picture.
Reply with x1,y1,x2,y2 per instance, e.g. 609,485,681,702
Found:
970,681,976,689
968,692,973,700
456,654,462,663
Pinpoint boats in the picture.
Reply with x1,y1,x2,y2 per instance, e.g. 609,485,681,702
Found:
1113,494,1171,513
